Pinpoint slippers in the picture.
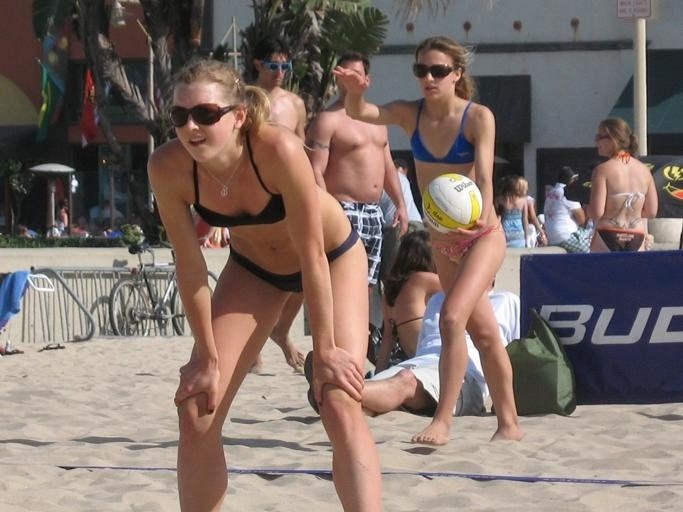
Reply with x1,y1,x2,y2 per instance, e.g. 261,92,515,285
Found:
1,350,24,355
43,344,65,350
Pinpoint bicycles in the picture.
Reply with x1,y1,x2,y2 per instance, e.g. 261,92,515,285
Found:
105,228,219,336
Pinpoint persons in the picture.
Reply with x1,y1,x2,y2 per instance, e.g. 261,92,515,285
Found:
248,45,521,416
191,206,231,248
16,198,124,240
147,60,382,512
497,117,659,253
331,37,523,446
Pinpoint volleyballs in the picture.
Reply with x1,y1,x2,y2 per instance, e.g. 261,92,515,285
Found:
422,173,483,234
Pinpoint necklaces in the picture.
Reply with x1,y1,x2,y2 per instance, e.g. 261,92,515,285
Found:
204,134,247,196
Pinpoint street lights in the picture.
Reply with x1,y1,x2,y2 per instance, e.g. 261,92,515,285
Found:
109,4,154,217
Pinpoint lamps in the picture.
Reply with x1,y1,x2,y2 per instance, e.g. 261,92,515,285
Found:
463,21,471,43
405,23,417,45
514,21,521,43
571,18,579,40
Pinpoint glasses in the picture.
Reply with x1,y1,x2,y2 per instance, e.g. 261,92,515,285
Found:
413,64,457,77
169,104,236,127
261,60,292,71
595,134,609,142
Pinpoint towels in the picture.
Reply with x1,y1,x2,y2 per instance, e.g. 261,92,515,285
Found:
0,271,30,330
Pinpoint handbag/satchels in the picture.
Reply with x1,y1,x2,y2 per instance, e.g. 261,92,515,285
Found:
491,308,576,416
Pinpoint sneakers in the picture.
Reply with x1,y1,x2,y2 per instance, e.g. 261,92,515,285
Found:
304,351,321,414
